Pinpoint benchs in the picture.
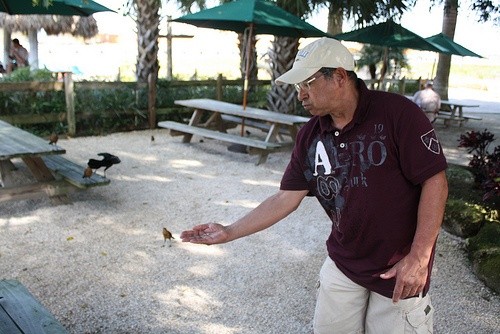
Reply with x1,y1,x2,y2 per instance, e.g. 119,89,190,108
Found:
41,154,110,190
158,121,281,152
0,277,70,334
220,114,300,136
438,111,481,127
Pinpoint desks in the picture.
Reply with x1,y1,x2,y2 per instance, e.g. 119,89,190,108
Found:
173,97,311,166
0,119,76,206
440,100,479,116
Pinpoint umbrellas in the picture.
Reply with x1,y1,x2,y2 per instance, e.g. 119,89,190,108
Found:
171,0,333,136
426,32,484,60
334,20,450,72
0,0,118,17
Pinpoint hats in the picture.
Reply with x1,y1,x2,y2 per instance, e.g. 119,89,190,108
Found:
275,36,356,85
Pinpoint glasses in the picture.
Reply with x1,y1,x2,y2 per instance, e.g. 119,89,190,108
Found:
293,69,338,92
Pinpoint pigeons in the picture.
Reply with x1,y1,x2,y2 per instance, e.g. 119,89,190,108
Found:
87,152,121,178
81,168,92,178
151,135,154,141
48,134,58,146
200,140,204,143
246,129,250,136
163,228,174,242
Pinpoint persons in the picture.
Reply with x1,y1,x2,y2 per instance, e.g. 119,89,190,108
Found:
180,37,449,334
6,40,28,74
412,80,441,123
